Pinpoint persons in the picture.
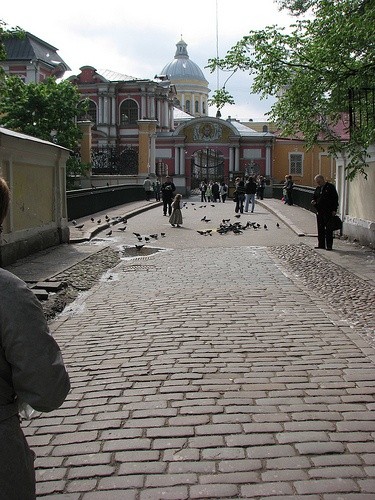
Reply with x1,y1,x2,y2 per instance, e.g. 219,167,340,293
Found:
310,173,339,250
160,176,176,217
153,177,162,203
143,176,152,201
0,173,70,500
199,172,295,213
168,193,183,228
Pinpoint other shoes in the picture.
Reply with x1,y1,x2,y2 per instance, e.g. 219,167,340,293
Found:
315,246,326,249
284,201,288,204
164,214,166,216
251,211,254,213
245,210,248,212
176,225,181,227
171,223,175,227
201,200,225,203
327,247,332,250
236,210,238,213
240,210,243,213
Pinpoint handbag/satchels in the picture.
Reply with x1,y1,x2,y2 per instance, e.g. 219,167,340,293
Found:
330,216,342,231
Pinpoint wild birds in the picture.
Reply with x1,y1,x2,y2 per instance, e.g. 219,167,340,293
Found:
118,227,126,232
135,245,144,251
75,224,84,229
91,215,127,225
183,202,279,236
72,220,77,225
106,230,112,237
133,232,166,243
